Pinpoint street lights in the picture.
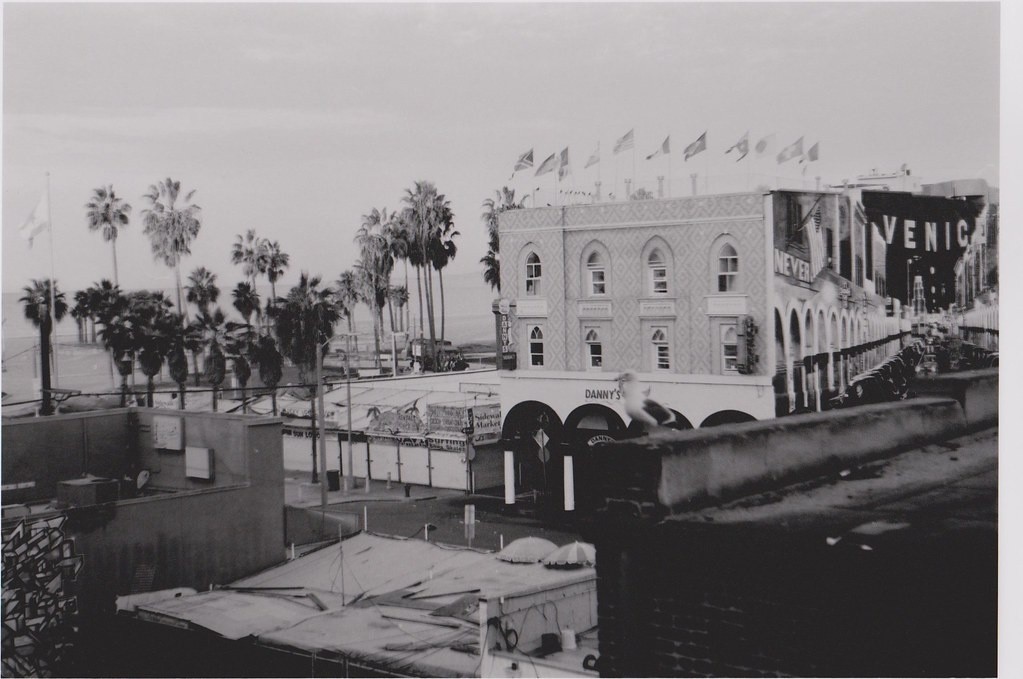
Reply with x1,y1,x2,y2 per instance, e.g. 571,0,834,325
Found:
316,332,363,509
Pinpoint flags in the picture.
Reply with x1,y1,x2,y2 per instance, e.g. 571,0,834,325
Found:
505,127,823,174
952,205,990,276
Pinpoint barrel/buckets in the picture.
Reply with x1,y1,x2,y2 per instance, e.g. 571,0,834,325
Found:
542,633,559,651
561,629,577,649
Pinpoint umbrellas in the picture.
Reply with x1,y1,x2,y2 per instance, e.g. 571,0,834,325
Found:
492,535,597,568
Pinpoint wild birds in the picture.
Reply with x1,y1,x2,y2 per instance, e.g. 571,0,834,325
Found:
613,369,678,431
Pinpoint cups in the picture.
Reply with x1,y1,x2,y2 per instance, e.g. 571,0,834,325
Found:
465,505,475,525
